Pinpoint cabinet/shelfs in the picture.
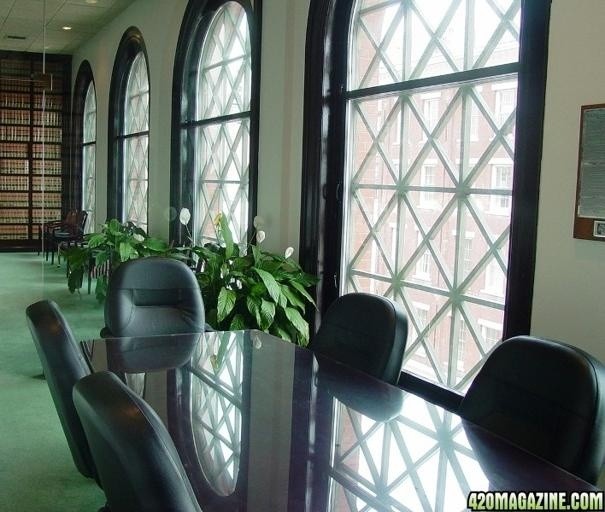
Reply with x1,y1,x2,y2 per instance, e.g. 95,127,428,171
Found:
0,49,74,253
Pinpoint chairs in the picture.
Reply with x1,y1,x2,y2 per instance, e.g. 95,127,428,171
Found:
24,204,605,512
